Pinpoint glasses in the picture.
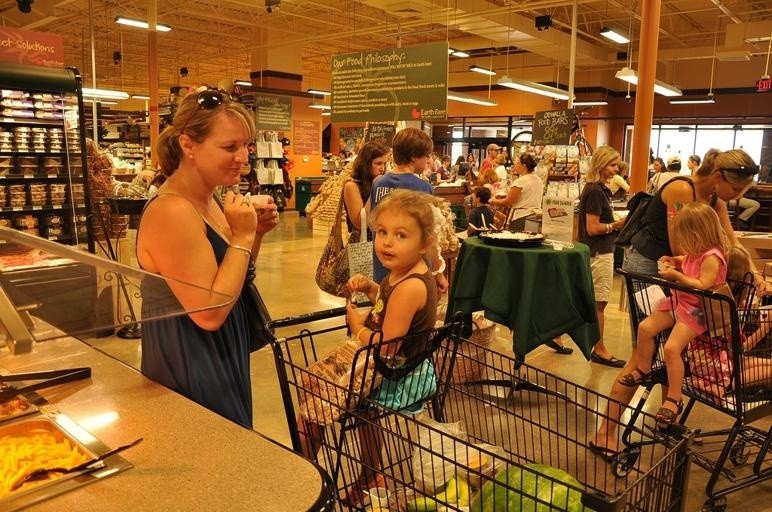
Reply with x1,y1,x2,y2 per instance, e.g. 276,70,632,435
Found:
715,164,760,178
179,89,234,134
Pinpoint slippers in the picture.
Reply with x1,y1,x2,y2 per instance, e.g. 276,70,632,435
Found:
589,441,635,465
655,422,703,443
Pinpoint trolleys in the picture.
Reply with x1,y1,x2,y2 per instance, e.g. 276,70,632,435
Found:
610,264,770,512
265,299,701,512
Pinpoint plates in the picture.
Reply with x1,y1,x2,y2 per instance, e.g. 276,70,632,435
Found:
478,231,545,246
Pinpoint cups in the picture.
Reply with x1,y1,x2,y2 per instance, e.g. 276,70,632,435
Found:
370,488,391,512
249,194,269,209
353,306,372,315
553,244,563,250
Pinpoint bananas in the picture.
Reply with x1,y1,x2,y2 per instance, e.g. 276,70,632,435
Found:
409,476,473,511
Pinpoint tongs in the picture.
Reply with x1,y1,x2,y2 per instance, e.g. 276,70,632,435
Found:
0,367,91,404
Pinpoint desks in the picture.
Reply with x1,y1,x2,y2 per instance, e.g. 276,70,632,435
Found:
445,233,600,369
1,315,334,512
295,177,325,217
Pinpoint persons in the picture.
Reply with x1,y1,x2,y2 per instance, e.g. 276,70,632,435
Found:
588,148,772,463
296,190,437,508
544,148,630,366
342,129,455,295
466,144,543,233
618,202,731,423
687,247,772,407
650,155,701,190
136,90,279,431
147,174,167,194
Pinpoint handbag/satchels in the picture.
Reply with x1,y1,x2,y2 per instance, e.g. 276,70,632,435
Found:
239,132,293,212
613,190,654,246
242,258,276,353
648,179,658,194
315,221,375,306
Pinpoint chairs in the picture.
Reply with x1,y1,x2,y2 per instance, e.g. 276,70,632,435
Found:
728,198,754,231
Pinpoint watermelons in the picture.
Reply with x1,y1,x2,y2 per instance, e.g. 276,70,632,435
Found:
472,465,585,512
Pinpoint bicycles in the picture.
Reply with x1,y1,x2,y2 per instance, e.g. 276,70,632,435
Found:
508,104,594,167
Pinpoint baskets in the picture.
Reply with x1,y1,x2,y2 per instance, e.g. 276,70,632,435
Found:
434,321,496,385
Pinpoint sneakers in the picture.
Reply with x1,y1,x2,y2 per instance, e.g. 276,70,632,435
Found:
739,221,749,228
591,350,626,367
545,338,573,354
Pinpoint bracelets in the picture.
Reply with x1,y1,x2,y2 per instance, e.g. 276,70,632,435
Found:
227,243,253,259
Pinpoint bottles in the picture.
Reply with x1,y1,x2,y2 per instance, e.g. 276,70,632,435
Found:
436,171,441,182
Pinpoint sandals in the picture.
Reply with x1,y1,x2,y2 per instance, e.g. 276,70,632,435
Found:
618,368,653,387
655,397,684,425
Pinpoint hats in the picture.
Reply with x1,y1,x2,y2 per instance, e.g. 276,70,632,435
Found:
668,157,681,166
487,144,502,150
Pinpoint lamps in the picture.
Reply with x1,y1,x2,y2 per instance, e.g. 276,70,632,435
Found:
668,10,719,105
114,14,170,33
447,49,498,107
615,13,682,96
235,80,250,86
81,87,148,105
497,1,576,101
599,2,630,45
307,88,331,115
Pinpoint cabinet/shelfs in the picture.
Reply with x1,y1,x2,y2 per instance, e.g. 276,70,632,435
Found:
83,108,153,213
0,62,93,253
